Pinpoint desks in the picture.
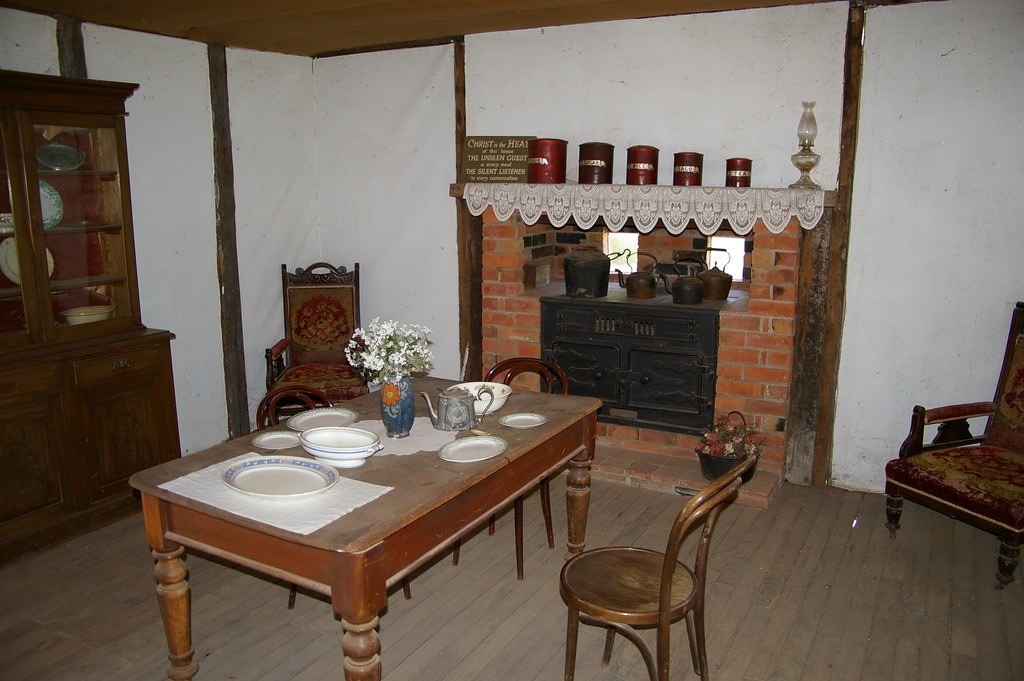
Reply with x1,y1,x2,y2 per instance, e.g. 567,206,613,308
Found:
128,377,607,680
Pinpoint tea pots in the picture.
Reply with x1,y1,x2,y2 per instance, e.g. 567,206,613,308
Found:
420,387,494,432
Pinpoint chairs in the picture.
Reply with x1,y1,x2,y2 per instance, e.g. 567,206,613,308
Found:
263,260,364,423
450,357,568,581
558,453,758,681
884,302,1024,590
255,384,411,611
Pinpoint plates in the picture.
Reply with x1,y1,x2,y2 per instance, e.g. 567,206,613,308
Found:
0,238,55,285
39,178,64,229
286,406,359,432
36,144,86,171
498,412,548,430
438,435,508,463
220,455,340,501
251,430,301,450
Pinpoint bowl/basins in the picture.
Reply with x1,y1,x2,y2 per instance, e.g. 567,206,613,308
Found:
0,214,14,234
297,427,384,469
446,381,512,416
61,306,114,325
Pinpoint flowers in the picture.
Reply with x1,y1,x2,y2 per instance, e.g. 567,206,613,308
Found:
695,415,761,460
345,316,435,381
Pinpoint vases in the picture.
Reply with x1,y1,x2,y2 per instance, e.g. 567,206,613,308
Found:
378,373,414,439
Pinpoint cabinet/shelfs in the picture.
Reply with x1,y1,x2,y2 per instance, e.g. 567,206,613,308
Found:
0,70,182,571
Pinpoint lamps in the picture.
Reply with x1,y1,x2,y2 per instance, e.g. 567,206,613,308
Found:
788,100,821,190
